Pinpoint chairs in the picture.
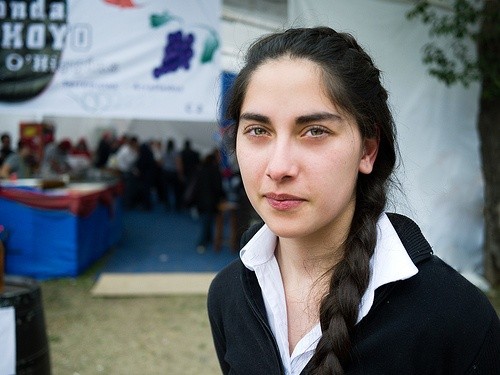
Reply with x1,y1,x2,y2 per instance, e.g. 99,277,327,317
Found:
212,200,242,252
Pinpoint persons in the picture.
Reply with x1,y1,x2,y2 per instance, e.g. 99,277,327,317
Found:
205,26,500,375
1,122,255,259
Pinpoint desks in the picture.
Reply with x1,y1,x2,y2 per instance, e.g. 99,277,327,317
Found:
1,175,128,279
